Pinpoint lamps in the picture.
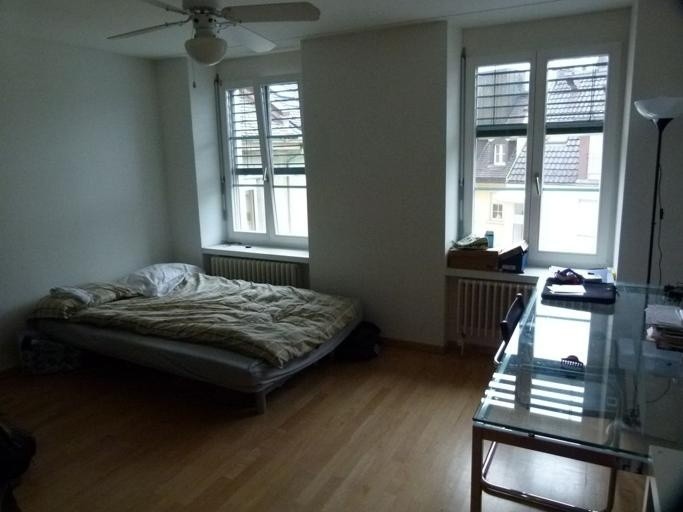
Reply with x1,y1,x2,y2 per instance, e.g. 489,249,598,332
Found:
601,95,683,449
183,14,227,66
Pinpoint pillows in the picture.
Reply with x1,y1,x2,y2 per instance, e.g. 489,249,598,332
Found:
25,262,204,320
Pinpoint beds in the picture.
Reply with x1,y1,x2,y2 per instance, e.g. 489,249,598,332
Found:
39,276,362,415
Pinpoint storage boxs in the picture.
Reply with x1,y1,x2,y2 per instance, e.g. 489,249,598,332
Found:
15,330,102,388
447,240,529,274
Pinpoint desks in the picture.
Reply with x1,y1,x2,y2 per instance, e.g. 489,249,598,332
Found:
469,270,683,511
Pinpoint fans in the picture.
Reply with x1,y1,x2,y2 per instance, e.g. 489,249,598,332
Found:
106,0,320,54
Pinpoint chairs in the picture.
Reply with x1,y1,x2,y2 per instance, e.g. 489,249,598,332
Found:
480,292,624,511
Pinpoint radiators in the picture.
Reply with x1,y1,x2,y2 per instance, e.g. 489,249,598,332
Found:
210,256,303,289
456,279,536,338
